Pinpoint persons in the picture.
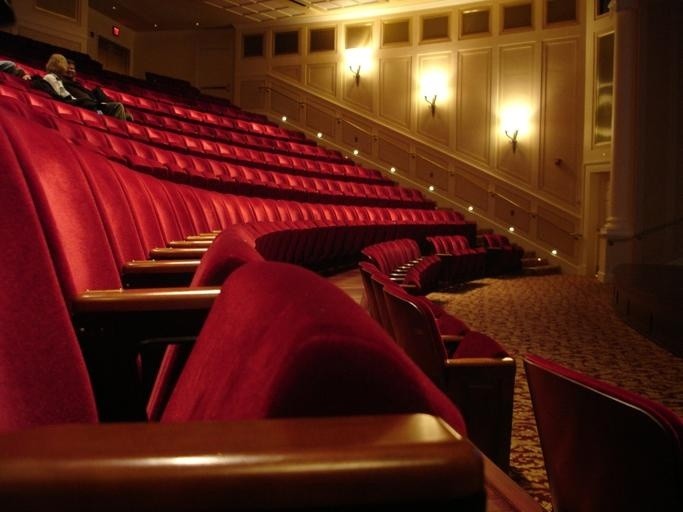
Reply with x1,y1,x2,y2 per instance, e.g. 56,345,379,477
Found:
61,57,133,120
1,0,30,80
42,53,94,106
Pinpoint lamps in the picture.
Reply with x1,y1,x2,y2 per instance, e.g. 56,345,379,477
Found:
343,46,373,84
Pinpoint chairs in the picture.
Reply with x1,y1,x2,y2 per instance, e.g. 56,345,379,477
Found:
518,350,680,512
0,32,523,512
